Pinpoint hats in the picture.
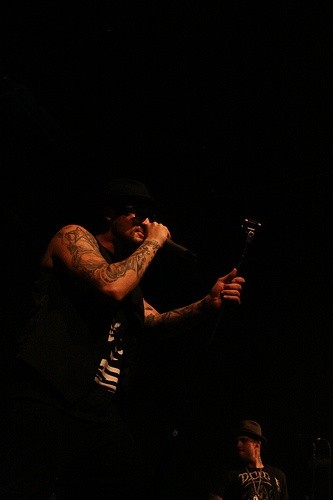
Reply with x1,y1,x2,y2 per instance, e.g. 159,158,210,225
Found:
235,420,267,447
94,180,156,217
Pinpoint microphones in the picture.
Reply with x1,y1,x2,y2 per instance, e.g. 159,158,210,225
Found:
166,239,195,258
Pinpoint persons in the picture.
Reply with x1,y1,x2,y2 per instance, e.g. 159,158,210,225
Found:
208,420,289,500
0,178,245,500
